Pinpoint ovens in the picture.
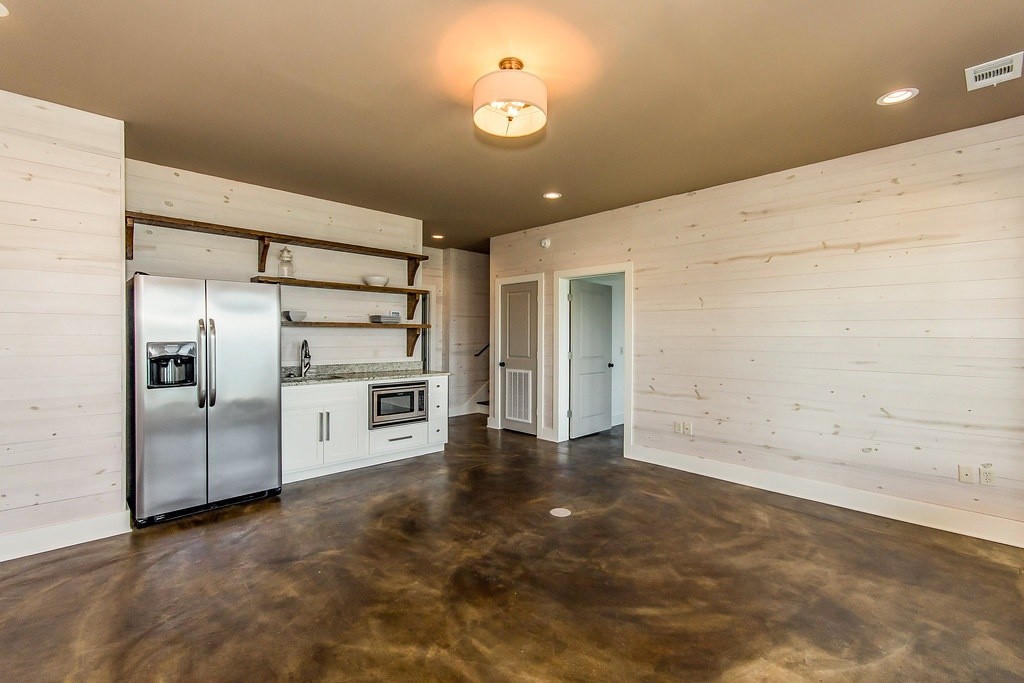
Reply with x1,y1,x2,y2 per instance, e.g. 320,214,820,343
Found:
367,379,429,430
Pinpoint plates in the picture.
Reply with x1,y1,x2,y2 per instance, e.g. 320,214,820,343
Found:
369,315,401,324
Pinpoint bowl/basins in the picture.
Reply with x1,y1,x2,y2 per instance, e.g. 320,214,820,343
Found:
364,276,389,287
283,310,307,322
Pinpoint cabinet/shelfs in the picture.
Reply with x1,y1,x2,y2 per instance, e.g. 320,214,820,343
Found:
280,373,450,486
124,210,430,358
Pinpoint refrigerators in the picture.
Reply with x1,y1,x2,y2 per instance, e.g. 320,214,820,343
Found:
125,269,282,527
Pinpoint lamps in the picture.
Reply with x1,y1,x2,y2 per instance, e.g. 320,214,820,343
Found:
471,56,549,140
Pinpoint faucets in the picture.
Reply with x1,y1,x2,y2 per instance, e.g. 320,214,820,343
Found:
299,339,311,378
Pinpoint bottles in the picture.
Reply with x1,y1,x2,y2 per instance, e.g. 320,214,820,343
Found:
278,246,297,277
